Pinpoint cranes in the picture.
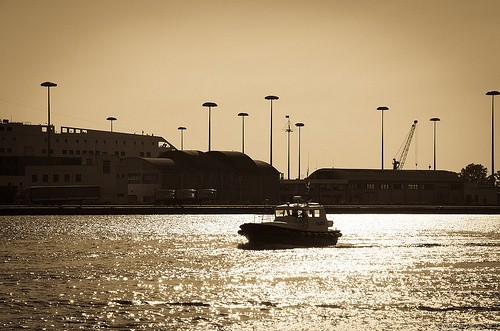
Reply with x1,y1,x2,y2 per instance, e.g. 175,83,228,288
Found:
390,119,418,170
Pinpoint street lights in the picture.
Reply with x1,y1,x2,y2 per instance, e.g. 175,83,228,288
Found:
265,95,280,167
430,117,440,171
486,90,500,178
178,126,186,151
295,122,304,181
376,106,389,171
202,102,218,152
238,112,249,155
107,117,117,132
40,82,58,166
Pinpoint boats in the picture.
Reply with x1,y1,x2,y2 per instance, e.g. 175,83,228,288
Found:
238,194,342,249
